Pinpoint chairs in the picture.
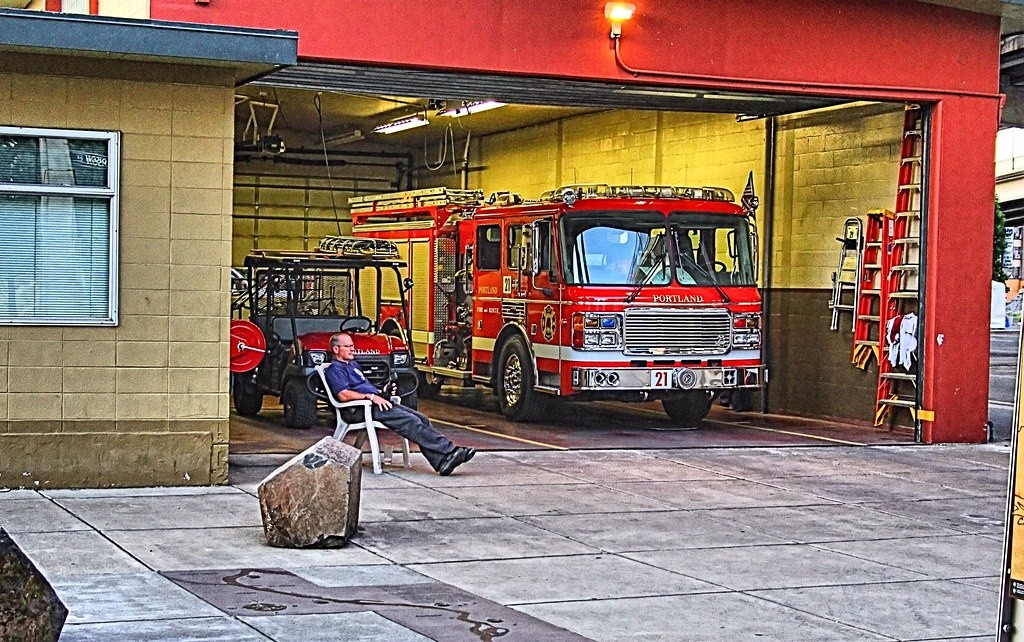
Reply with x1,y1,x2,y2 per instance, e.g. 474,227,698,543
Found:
655,233,695,268
315,363,411,474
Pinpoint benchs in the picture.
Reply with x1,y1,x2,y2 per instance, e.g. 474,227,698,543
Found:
258,315,372,340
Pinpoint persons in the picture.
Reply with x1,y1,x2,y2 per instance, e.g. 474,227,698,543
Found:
324,333,476,476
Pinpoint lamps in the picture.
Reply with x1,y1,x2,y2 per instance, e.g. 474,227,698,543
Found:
316,130,364,146
435,101,508,117
374,113,430,135
604,2,636,37
234,136,286,159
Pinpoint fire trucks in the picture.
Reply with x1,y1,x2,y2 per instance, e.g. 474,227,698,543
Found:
349,183,769,425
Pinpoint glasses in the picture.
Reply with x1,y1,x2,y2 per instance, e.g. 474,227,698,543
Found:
336,343,354,349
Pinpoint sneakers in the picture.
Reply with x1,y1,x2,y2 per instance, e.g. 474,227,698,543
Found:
443,445,475,461
439,448,465,476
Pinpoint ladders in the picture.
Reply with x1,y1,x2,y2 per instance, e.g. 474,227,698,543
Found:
849,207,896,366
873,101,933,444
829,215,864,333
